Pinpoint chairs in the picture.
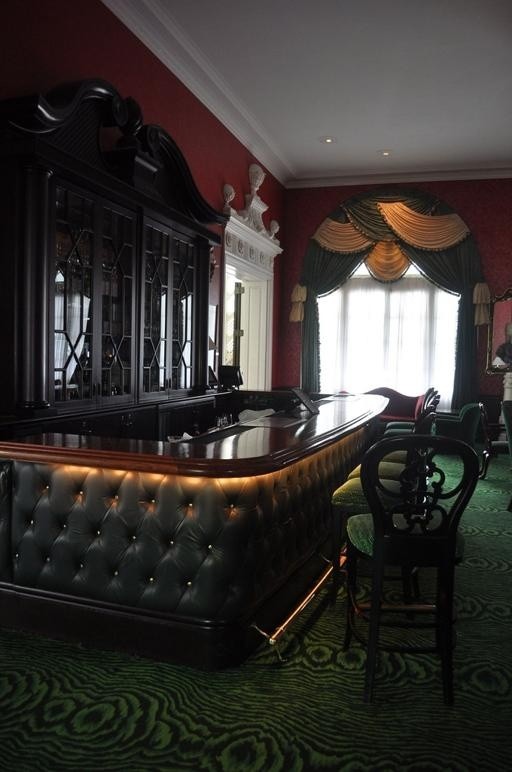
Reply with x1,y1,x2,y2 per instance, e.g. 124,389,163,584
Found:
434,401,479,455
343,435,478,708
329,387,441,619
478,402,510,480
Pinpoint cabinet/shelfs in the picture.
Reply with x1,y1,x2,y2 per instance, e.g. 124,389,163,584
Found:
0,71,232,444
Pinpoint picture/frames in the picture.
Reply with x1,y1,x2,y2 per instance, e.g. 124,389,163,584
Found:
485,288,512,376
208,364,218,383
285,387,320,416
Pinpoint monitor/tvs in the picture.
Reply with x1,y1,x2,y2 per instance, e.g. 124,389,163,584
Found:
220,366,243,389
